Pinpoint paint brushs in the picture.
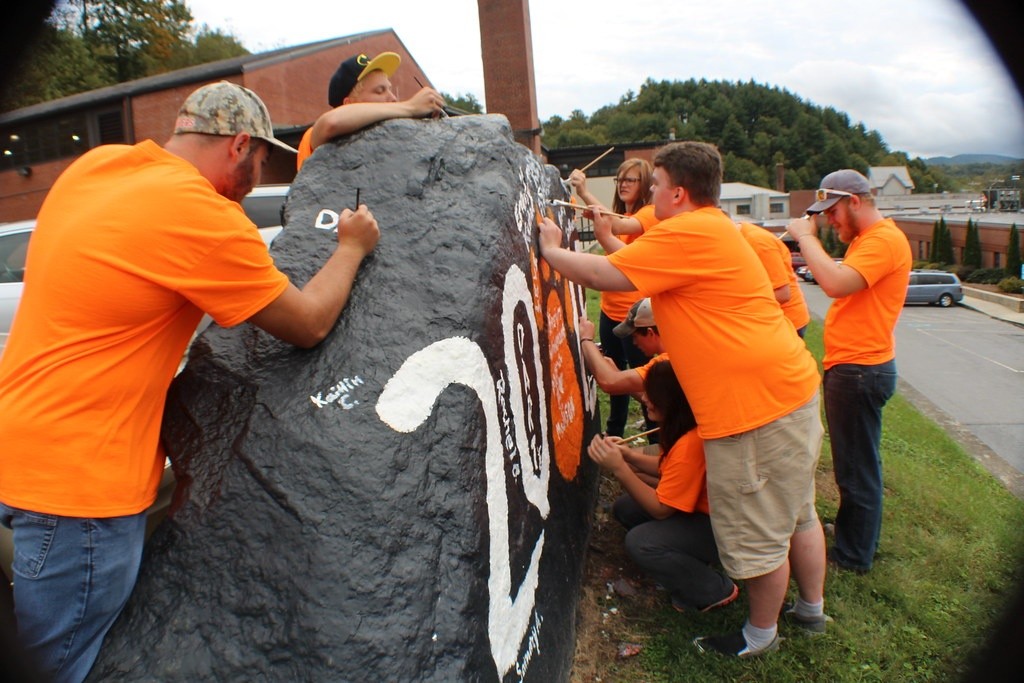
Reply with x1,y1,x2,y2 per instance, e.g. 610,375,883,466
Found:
550,199,630,219
413,74,449,118
562,146,614,185
777,214,810,240
580,303,587,368
614,427,659,444
356,188,360,211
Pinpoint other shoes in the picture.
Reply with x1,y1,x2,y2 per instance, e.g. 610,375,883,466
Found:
826,547,867,576
779,604,826,637
823,522,836,539
692,625,780,660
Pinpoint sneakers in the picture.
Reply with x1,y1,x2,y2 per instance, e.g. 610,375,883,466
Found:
673,583,739,614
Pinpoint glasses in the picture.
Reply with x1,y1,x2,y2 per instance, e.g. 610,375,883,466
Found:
613,178,641,186
815,188,854,202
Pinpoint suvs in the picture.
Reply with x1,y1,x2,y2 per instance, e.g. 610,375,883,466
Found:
904,269,963,308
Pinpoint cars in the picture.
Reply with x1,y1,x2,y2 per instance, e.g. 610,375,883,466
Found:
790,251,844,283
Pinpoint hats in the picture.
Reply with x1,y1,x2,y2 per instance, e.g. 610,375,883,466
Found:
613,297,659,337
173,79,299,153
806,169,870,217
328,51,401,108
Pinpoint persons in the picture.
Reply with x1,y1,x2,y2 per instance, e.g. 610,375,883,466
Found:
785,169,912,576
537,142,828,658
296,50,448,170
0,80,379,683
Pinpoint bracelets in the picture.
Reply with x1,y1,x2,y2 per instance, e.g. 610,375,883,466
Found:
580,338,593,345
796,234,816,243
